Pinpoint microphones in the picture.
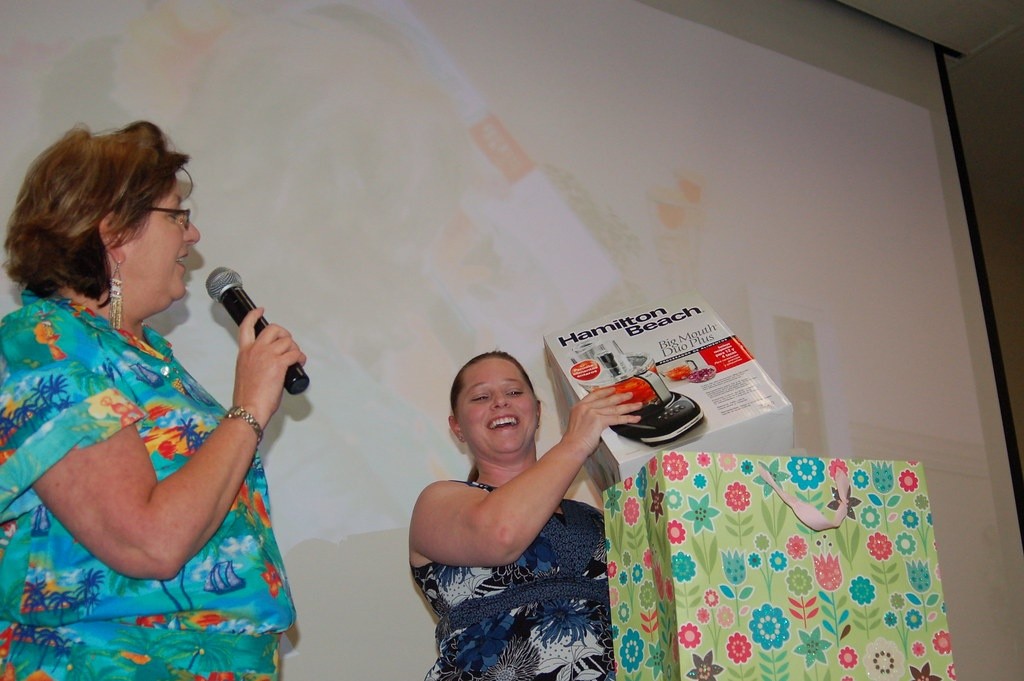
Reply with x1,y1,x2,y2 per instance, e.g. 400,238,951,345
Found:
205,267,310,395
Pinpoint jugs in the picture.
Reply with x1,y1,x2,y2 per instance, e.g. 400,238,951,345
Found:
658,359,698,381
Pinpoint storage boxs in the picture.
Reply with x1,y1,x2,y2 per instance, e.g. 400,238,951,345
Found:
542,290,793,500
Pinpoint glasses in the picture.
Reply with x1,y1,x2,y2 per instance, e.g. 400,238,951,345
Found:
149,207,190,227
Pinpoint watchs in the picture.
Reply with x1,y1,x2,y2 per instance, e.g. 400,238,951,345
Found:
226,407,264,442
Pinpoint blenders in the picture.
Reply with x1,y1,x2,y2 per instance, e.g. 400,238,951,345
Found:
570,338,705,447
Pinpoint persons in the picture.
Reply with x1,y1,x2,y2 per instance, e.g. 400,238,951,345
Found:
409,352,643,681
0,120,305,681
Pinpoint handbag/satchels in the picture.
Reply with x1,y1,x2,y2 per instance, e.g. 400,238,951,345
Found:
600,450,955,681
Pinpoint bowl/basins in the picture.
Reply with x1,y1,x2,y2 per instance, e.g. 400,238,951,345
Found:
688,365,716,384
